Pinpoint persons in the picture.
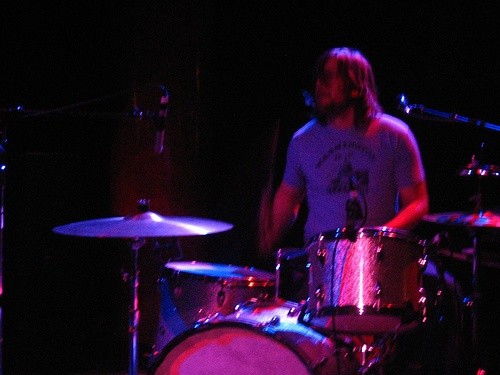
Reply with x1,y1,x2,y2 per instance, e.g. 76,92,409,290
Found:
259,45,431,254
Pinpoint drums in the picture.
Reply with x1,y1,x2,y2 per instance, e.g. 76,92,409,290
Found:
150,298,356,375
149,261,277,365
303,226,427,336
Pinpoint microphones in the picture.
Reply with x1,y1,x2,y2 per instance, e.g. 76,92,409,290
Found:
154,90,168,154
399,94,411,116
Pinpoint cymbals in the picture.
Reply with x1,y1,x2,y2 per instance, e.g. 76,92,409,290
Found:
421,210,500,229
52,212,233,237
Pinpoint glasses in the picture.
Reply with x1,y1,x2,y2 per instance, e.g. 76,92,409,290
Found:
316,70,343,83
